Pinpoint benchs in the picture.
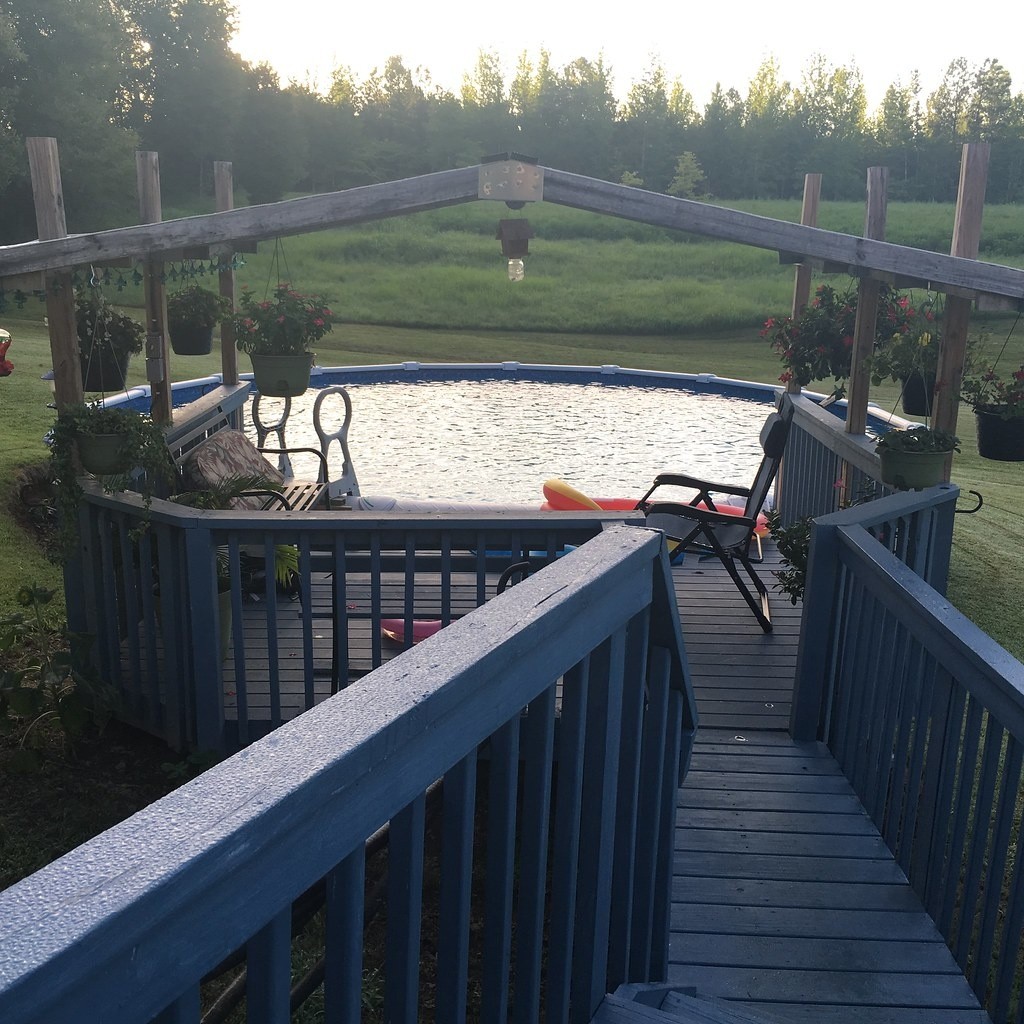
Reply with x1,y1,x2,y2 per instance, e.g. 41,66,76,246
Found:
164,404,329,605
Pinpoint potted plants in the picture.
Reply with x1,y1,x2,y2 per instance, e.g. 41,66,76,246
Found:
50,402,302,655
870,426,961,487
167,283,232,355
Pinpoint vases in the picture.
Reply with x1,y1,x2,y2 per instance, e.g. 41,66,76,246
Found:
249,352,316,397
830,354,852,377
81,350,131,392
975,405,1024,461
899,373,937,416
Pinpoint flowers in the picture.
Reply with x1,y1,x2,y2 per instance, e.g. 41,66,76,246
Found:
761,285,941,385
959,369,1024,421
235,282,335,355
44,292,147,359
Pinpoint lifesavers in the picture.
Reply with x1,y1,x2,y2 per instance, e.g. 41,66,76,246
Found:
378,617,454,645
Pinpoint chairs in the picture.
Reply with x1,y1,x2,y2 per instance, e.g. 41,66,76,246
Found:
638,392,793,633
